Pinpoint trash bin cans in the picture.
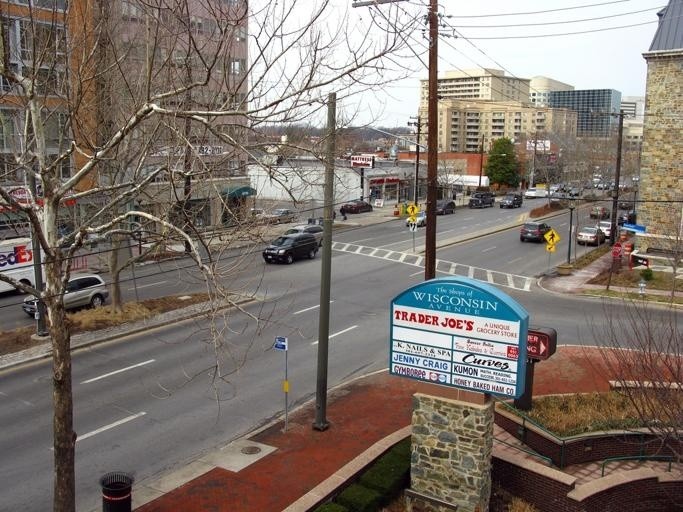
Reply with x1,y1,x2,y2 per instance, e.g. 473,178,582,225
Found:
99,471,135,512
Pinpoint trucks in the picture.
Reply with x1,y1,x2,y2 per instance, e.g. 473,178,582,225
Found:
0,237,46,295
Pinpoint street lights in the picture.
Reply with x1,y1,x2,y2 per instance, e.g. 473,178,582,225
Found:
566,193,576,264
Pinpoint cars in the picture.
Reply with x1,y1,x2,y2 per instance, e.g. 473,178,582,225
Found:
341,200,373,214
22,272,110,319
262,225,324,264
251,207,297,225
406,164,640,247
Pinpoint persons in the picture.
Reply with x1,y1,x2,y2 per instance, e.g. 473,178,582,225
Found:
339,205,345,220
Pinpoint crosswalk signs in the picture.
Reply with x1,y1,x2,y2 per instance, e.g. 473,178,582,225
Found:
544,225,562,255
